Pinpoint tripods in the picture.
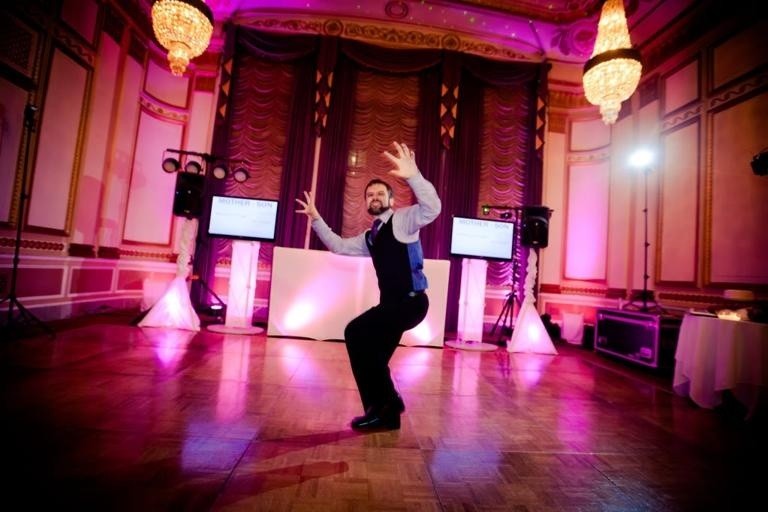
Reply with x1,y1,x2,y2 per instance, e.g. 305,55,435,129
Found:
130,220,225,327
620,178,671,315
491,213,523,344
0,126,53,334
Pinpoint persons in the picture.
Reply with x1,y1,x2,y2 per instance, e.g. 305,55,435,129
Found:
293,139,443,434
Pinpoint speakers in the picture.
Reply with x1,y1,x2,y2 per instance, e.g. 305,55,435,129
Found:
173,171,205,218
520,207,549,248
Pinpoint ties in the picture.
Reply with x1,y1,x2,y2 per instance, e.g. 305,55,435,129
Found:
370,218,381,245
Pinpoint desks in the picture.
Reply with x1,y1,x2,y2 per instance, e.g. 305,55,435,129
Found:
673,306,768,478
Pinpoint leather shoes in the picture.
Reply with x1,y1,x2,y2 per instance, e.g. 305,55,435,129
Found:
350,399,405,431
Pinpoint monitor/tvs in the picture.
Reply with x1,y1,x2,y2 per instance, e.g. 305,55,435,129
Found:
207,194,280,242
449,215,517,262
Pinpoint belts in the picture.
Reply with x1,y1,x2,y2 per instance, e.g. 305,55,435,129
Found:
406,289,425,297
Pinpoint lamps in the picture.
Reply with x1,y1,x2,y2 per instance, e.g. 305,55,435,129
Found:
581,0,645,126
479,203,554,344
161,147,250,330
148,0,217,78
